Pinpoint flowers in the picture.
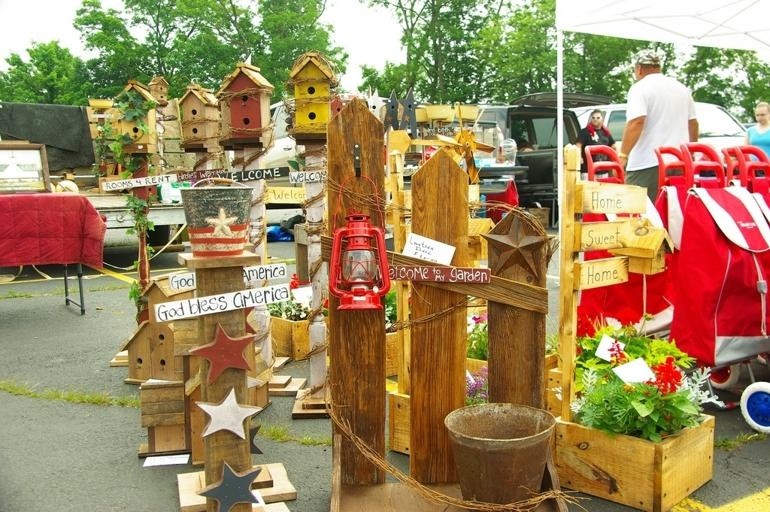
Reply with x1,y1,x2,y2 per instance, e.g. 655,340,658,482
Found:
465,366,490,406
465,309,489,361
547,312,720,441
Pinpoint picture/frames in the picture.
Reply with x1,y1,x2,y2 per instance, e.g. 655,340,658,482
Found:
0,143,51,193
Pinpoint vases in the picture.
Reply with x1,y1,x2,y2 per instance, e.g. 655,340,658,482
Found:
556,415,715,511
466,358,489,374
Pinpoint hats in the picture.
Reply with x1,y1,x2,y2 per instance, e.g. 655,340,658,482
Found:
633,49,660,66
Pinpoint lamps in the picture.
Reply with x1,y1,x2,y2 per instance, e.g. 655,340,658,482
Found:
327,174,391,311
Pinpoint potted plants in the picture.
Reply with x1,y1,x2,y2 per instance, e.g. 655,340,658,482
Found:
267,292,312,363
384,289,401,378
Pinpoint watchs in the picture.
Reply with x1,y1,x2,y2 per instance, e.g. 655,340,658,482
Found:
617,152,628,159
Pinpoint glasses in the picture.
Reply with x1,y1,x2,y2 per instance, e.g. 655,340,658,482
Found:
591,117,601,120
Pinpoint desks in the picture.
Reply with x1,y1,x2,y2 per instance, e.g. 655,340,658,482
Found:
1,192,89,315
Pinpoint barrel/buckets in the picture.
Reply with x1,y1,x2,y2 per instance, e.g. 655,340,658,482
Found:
179,187,253,258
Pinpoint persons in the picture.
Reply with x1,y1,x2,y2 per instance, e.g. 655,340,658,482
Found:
743,101,770,176
574,109,616,181
617,48,698,203
